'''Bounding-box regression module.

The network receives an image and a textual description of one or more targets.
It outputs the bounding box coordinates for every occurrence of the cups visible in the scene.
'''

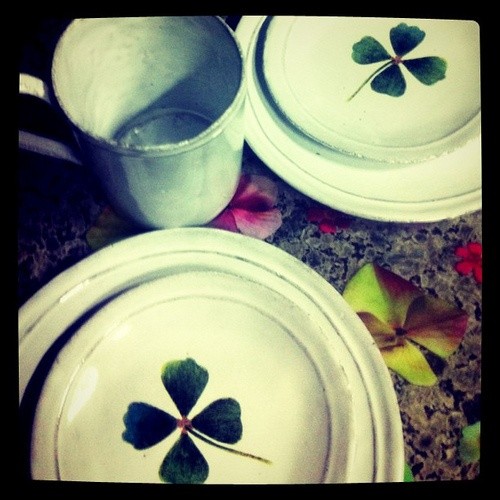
[16,18,247,230]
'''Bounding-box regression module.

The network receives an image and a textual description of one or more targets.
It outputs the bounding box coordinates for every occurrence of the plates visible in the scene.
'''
[17,227,406,484]
[235,16,481,225]
